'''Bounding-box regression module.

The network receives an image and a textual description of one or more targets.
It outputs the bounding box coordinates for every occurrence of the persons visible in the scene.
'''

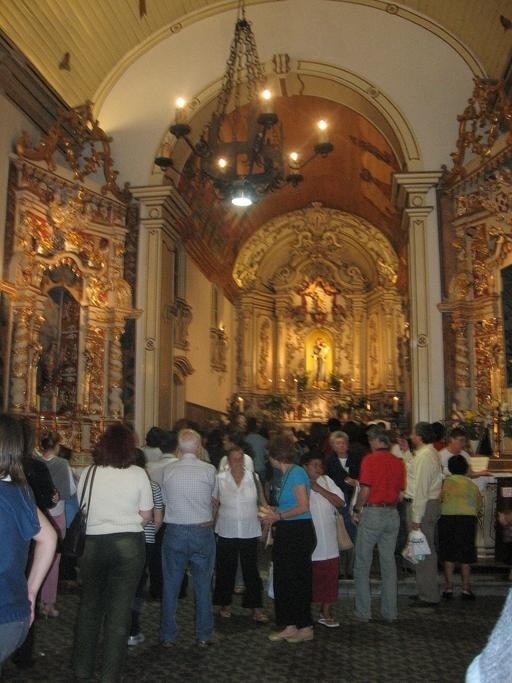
[309,337,329,381]
[3,412,511,682]
[317,340,329,362]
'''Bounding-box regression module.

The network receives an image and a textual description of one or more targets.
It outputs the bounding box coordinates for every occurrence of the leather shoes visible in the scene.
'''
[286,624,314,643]
[408,599,441,608]
[268,628,298,641]
[409,594,419,600]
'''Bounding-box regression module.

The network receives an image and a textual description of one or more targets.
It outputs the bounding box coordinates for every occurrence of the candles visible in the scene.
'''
[237,378,400,414]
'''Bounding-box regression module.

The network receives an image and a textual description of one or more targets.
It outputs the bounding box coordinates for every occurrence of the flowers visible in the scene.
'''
[461,410,479,439]
[498,402,511,437]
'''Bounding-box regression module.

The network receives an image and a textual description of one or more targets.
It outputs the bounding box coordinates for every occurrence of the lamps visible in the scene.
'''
[149,0,337,211]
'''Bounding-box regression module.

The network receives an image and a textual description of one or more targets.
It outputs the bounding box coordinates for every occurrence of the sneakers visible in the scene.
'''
[127,632,145,645]
[160,638,176,647]
[195,637,209,649]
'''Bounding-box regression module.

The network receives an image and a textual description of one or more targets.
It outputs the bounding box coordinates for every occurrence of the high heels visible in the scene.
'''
[39,602,59,623]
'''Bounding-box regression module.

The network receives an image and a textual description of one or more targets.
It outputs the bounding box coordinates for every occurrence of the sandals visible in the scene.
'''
[443,586,454,597]
[462,588,474,599]
[252,613,269,621]
[219,608,232,617]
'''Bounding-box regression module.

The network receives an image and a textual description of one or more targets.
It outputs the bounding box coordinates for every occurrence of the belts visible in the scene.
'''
[363,502,397,507]
[405,497,412,502]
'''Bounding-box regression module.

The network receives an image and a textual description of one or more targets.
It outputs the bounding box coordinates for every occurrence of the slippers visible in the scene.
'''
[317,617,341,627]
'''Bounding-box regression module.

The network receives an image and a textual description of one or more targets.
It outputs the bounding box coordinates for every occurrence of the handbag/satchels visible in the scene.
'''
[251,471,272,542]
[60,463,97,557]
[335,512,354,551]
